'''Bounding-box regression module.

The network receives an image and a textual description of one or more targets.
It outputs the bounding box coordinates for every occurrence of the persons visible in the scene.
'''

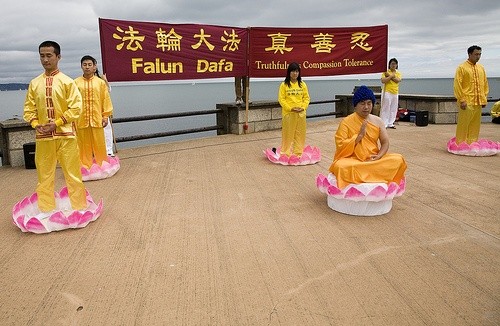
[454,46,489,146]
[329,85,408,189]
[74,55,115,171]
[380,58,402,128]
[490,101,500,124]
[23,40,87,221]
[278,62,310,158]
[235,77,252,105]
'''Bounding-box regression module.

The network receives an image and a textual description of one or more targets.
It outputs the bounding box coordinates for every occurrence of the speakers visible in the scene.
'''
[23,142,36,169]
[415,110,428,126]
